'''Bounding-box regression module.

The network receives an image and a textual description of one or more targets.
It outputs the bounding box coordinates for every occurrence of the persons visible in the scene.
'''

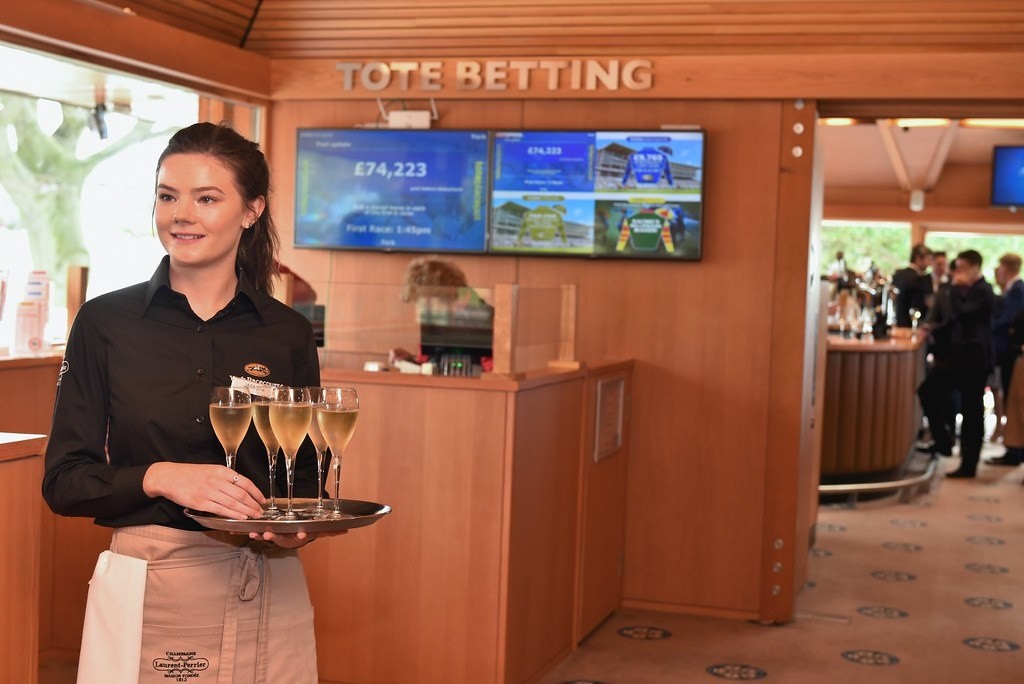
[389,258,494,373]
[892,245,1024,479]
[42,122,348,684]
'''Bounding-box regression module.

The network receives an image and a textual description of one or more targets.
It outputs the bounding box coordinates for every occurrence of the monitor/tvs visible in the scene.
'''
[293,125,702,259]
[990,146,1024,208]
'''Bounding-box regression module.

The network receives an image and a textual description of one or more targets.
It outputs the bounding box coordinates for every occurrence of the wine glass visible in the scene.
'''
[208,384,360,521]
[910,307,921,330]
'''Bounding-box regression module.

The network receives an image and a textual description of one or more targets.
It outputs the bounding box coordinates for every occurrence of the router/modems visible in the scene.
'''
[376,94,438,128]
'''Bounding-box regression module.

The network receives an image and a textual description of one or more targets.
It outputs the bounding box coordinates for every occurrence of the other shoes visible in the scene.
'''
[946,469,977,478]
[984,455,1021,466]
[914,444,953,457]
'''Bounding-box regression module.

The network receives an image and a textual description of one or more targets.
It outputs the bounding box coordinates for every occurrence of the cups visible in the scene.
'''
[839,318,863,339]
